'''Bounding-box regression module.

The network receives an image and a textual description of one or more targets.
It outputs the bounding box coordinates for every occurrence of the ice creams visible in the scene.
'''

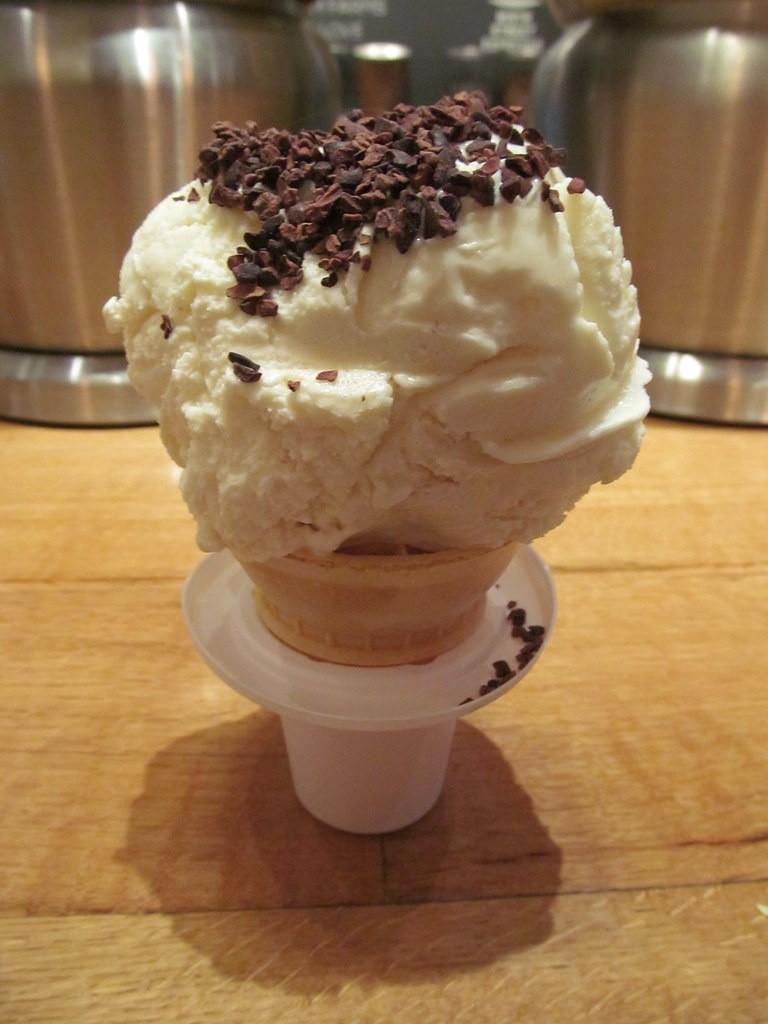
[101,89,650,668]
